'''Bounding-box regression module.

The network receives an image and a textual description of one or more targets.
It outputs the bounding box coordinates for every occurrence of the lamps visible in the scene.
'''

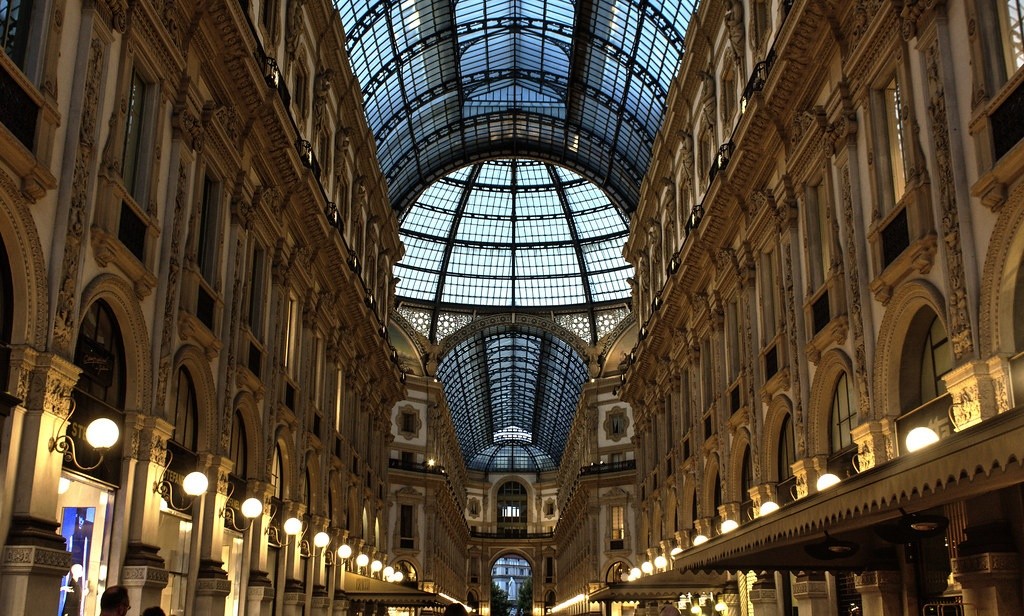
[219,471,263,531]
[906,394,967,453]
[590,461,605,466]
[720,502,757,533]
[761,479,802,515]
[48,387,118,470]
[296,521,329,557]
[323,537,352,566]
[816,446,868,490]
[259,57,404,380]
[262,499,302,547]
[370,560,382,579]
[345,553,368,576]
[611,82,748,396]
[152,434,208,510]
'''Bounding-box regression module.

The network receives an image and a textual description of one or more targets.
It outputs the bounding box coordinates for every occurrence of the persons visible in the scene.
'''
[142,606,166,616]
[508,576,515,596]
[98,584,131,616]
[61,563,83,616]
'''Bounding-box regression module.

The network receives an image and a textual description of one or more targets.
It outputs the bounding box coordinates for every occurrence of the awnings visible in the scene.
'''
[337,572,456,609]
[673,402,1022,575]
[584,570,732,604]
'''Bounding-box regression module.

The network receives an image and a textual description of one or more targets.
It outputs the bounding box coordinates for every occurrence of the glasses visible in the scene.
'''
[127,606,131,610]
